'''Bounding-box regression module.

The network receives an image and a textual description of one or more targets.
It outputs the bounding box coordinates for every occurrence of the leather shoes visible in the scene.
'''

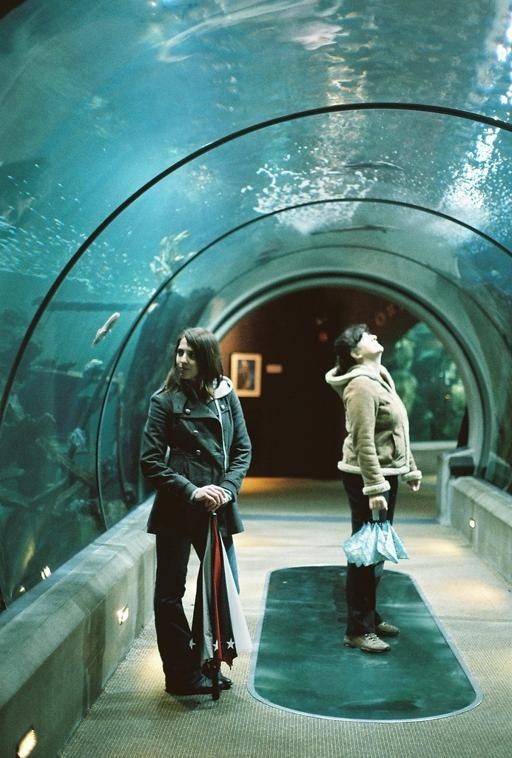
[166,666,232,693]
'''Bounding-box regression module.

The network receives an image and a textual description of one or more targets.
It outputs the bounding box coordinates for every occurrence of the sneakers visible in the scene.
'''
[374,622,400,637]
[344,631,391,653]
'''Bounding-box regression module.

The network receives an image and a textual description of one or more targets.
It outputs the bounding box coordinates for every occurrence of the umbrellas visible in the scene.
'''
[188,510,252,699]
[341,508,410,568]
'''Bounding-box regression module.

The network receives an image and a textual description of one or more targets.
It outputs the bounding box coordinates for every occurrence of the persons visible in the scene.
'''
[325,323,422,653]
[138,327,253,695]
